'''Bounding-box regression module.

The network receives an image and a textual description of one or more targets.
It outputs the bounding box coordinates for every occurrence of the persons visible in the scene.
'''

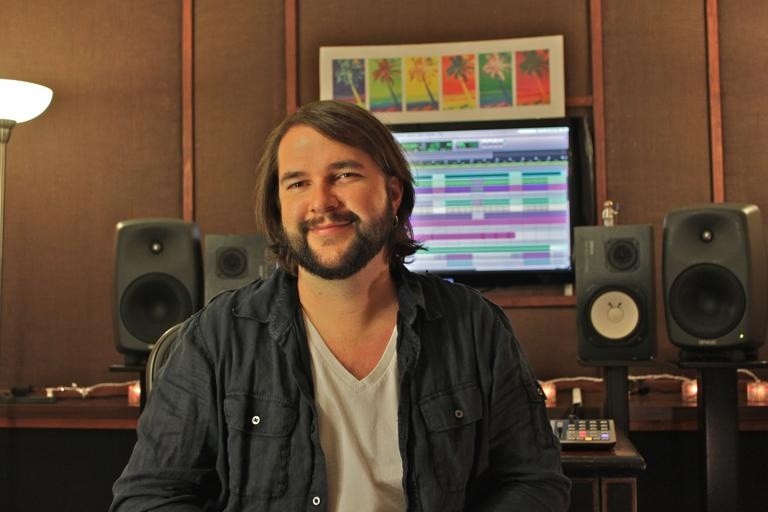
[108,101,572,511]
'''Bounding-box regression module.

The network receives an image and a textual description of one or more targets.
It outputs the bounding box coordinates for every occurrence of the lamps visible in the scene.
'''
[1,78,53,272]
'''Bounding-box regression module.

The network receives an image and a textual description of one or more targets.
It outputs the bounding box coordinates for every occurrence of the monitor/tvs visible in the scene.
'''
[382,118,585,287]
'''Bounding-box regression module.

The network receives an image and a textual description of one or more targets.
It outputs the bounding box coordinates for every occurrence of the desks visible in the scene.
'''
[1,374,768,511]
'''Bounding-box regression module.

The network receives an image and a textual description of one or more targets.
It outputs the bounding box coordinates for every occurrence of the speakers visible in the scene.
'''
[108,219,202,372]
[202,234,268,312]
[574,225,658,366]
[662,203,768,368]
